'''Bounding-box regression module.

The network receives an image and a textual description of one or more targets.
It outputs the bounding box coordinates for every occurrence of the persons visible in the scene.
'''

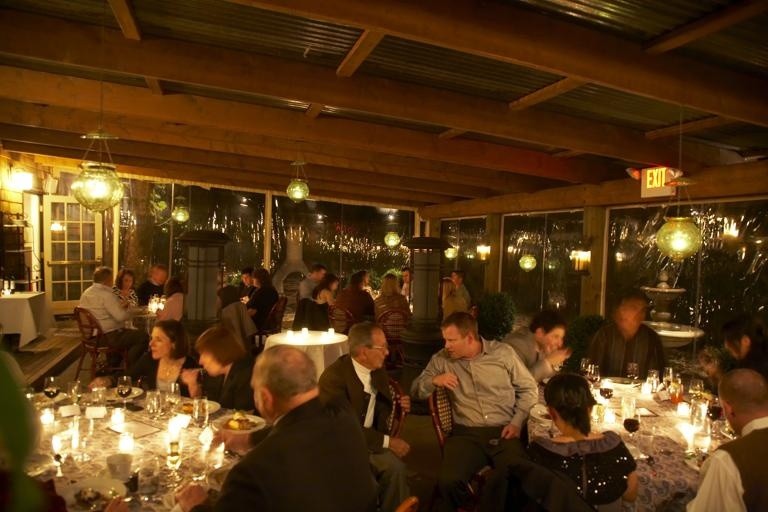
[241,269,279,331]
[441,277,467,318]
[306,274,340,331]
[217,286,257,336]
[373,273,411,320]
[127,318,201,398]
[78,265,152,380]
[712,319,766,378]
[504,309,572,383]
[237,268,253,300]
[408,311,538,511]
[451,271,472,307]
[685,368,768,512]
[528,373,635,511]
[173,343,376,512]
[318,321,410,511]
[114,269,139,306]
[299,264,327,300]
[154,277,184,321]
[358,270,373,295]
[586,289,665,381]
[401,268,411,302]
[331,274,374,336]
[194,325,254,410]
[137,265,168,306]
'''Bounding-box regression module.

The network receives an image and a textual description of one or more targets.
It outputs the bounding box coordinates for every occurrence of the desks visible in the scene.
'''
[263,329,349,384]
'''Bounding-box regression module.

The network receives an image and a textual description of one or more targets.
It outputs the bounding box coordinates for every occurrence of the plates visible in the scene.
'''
[529,405,555,424]
[36,390,67,408]
[101,385,143,404]
[212,413,267,436]
[172,400,221,418]
[64,477,128,512]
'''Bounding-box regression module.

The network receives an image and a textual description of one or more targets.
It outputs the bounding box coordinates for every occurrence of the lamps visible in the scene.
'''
[169,188,191,225]
[384,222,401,249]
[71,21,124,214]
[285,145,310,203]
[517,249,537,272]
[568,240,592,276]
[445,246,457,262]
[476,243,491,265]
[656,108,702,266]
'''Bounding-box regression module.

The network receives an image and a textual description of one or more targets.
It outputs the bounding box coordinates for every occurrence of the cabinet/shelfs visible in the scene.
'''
[0,211,44,292]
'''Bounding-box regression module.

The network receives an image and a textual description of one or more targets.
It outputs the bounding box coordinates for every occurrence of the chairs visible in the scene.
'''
[478,457,597,512]
[252,296,288,352]
[292,298,329,331]
[427,385,537,511]
[376,309,411,368]
[384,374,407,439]
[73,307,128,388]
[219,302,264,358]
[391,495,419,512]
[327,304,355,335]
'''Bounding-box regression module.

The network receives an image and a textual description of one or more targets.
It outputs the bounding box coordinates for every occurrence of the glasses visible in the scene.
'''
[365,342,388,349]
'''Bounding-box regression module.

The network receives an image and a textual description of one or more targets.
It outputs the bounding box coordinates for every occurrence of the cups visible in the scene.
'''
[641,383,651,399]
[91,386,106,409]
[621,397,636,420]
[189,446,206,480]
[39,407,54,430]
[165,437,184,483]
[106,454,133,482]
[111,408,126,425]
[118,432,133,451]
[637,433,655,460]
[138,457,161,499]
[669,384,683,409]
[647,369,660,393]
[676,402,690,417]
[203,441,225,472]
[689,379,704,398]
[146,390,162,419]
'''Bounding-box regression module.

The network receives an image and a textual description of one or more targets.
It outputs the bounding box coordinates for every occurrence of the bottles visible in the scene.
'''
[0,267,17,295]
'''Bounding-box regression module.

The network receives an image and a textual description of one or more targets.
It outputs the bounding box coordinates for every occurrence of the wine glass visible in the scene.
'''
[580,358,593,377]
[663,368,674,391]
[599,378,614,401]
[117,375,133,413]
[627,363,640,387]
[693,433,711,471]
[192,396,210,436]
[72,416,95,462]
[166,383,181,415]
[625,411,640,449]
[51,435,69,479]
[707,397,723,435]
[44,376,61,411]
[68,380,83,407]
[585,365,600,396]
[149,294,167,313]
